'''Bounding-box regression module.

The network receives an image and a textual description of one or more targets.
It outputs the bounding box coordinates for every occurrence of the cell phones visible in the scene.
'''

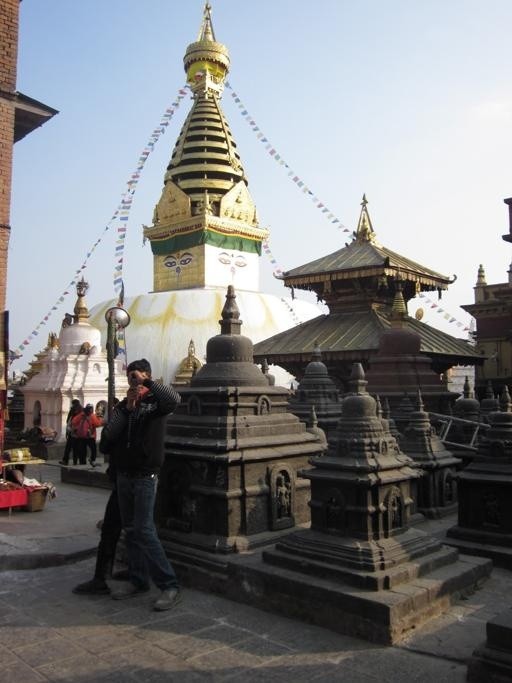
[129,376,138,388]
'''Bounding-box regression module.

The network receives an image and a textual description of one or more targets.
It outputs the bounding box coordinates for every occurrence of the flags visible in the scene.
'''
[222,76,478,339]
[9,73,194,372]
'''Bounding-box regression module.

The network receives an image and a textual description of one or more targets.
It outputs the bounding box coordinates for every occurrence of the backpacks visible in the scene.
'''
[76,415,92,438]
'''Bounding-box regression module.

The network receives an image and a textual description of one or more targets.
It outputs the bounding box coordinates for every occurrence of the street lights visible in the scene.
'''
[104,306,131,424]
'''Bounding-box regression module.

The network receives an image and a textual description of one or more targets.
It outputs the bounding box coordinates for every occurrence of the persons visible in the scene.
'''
[327,496,338,527]
[68,425,131,595]
[275,474,290,518]
[99,357,183,611]
[189,402,197,414]
[57,398,82,465]
[261,400,268,414]
[71,402,104,467]
[391,497,400,524]
[445,483,452,500]
[177,337,202,372]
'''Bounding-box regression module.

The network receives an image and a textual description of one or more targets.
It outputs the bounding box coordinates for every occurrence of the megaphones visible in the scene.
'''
[104,307,132,328]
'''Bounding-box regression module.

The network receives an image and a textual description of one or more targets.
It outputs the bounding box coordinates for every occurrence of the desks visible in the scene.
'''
[1,455,47,515]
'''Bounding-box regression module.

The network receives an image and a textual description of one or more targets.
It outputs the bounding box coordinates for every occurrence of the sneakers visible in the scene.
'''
[153,589,183,611]
[88,458,95,467]
[59,461,68,465]
[72,580,114,594]
[113,570,130,582]
[111,585,149,600]
